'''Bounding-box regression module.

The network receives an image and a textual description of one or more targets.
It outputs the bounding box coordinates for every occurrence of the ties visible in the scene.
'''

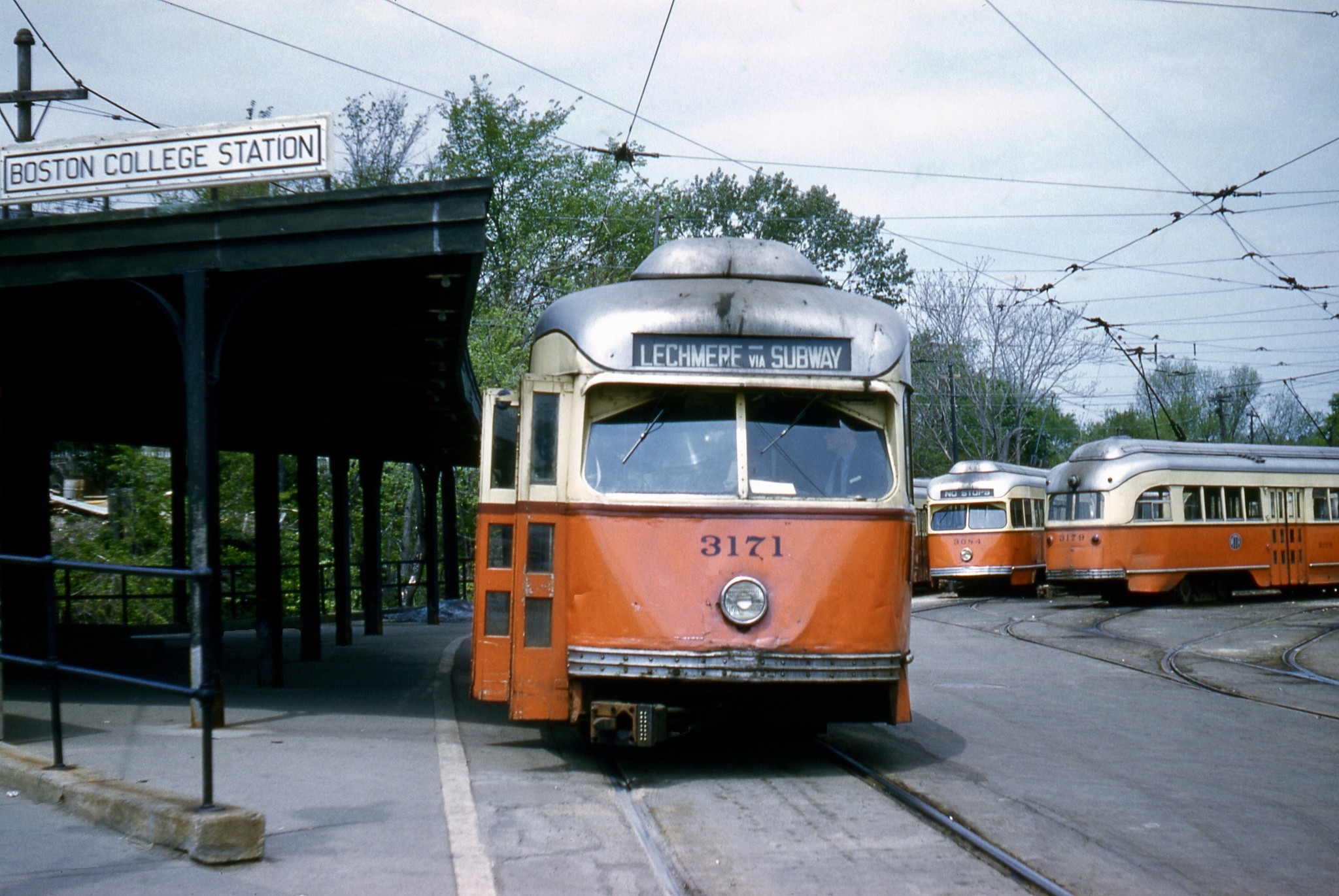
[832,459,842,496]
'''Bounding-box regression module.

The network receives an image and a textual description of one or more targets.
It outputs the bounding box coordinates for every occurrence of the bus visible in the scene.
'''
[913,478,943,593]
[469,235,917,757]
[1043,435,1339,606]
[926,460,1093,598]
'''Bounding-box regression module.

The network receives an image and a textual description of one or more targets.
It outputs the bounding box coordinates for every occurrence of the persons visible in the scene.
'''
[807,415,890,497]
